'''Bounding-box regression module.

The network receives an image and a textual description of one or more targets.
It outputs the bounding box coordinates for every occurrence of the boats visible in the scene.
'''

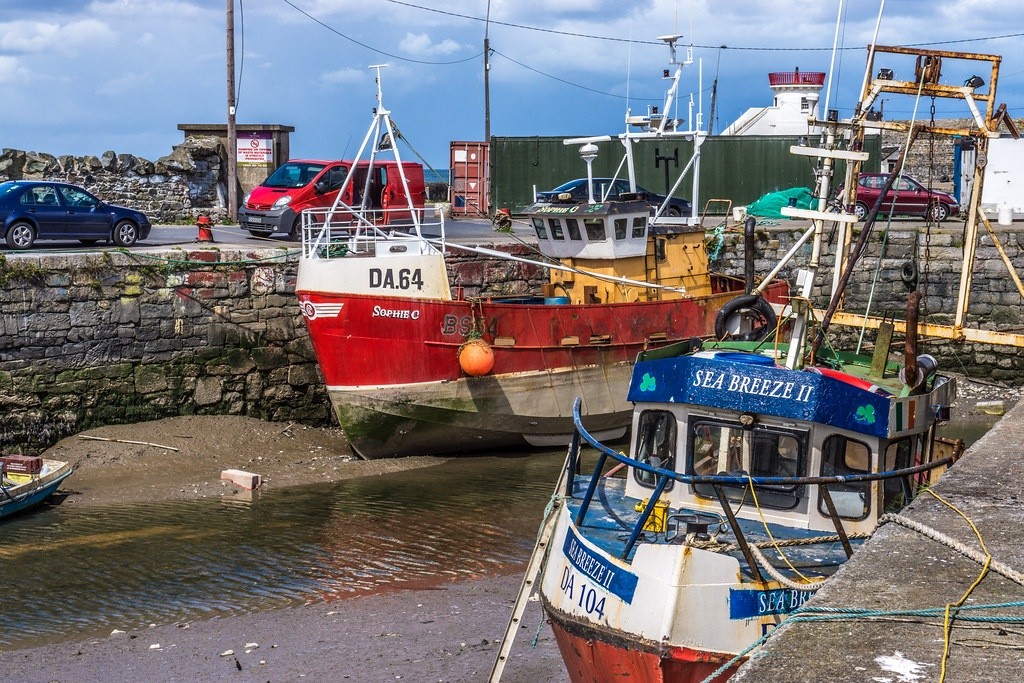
[0,455,74,521]
[489,107,965,683]
[295,58,791,460]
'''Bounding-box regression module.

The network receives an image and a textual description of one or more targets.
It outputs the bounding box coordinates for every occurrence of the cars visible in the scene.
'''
[536,178,693,218]
[0,180,153,253]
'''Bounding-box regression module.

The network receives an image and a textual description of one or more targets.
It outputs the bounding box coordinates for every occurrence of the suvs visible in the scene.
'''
[836,172,961,222]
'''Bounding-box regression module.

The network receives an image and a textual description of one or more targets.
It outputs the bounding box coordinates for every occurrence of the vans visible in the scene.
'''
[238,158,425,242]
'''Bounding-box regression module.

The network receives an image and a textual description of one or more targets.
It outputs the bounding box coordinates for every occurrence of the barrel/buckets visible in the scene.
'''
[545,297,568,304]
